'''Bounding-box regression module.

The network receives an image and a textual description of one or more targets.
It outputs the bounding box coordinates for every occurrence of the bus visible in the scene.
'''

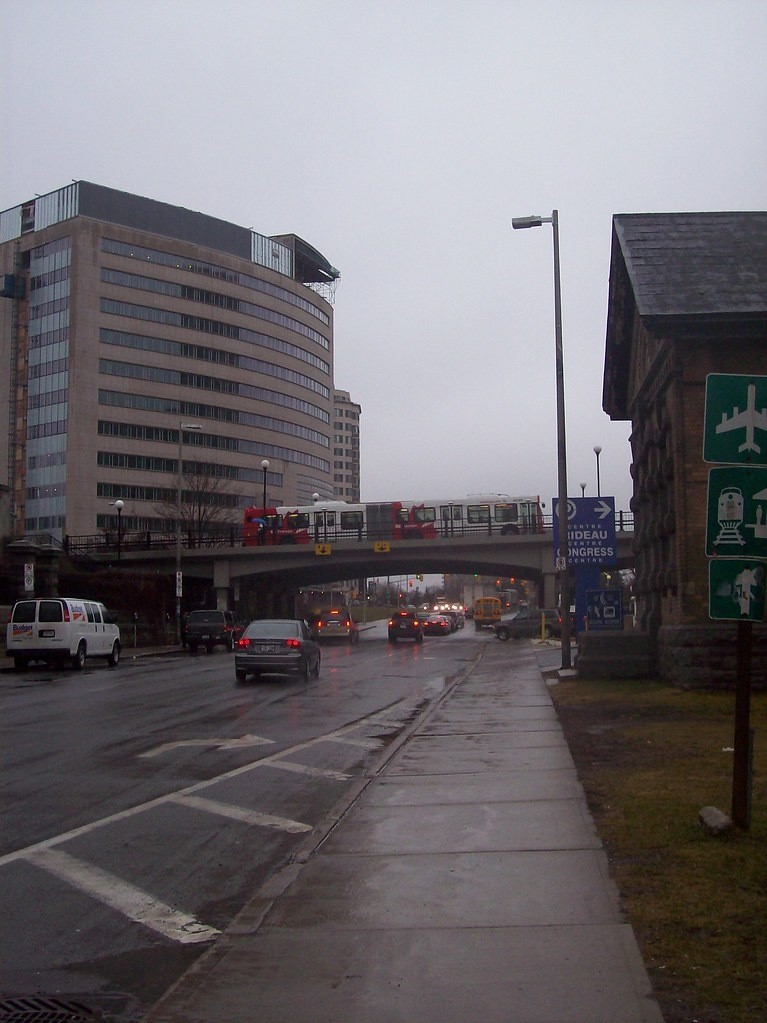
[242,494,545,549]
[475,597,503,629]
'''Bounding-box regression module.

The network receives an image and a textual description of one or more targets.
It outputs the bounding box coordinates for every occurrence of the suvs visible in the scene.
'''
[186,609,239,655]
[388,610,425,643]
[492,608,575,642]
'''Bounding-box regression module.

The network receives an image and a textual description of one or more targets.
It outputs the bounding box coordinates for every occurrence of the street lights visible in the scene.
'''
[259,458,271,509]
[173,421,204,641]
[511,210,571,667]
[115,500,125,568]
[580,482,587,498]
[593,445,603,500]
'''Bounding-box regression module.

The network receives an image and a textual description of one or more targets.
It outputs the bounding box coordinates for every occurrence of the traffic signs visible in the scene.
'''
[553,496,616,575]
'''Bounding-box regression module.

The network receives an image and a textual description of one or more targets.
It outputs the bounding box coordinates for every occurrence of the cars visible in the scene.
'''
[316,609,359,643]
[235,619,322,683]
[408,602,465,630]
[423,614,451,635]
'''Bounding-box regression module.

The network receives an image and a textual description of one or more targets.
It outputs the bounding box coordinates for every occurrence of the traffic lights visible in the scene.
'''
[524,588,529,596]
[474,574,480,581]
[416,574,421,579]
[409,581,412,587]
[399,593,402,598]
[510,578,515,585]
[421,576,423,582]
[496,580,501,588]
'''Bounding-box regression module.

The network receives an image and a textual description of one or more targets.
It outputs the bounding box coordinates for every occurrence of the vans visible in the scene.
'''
[5,597,123,673]
